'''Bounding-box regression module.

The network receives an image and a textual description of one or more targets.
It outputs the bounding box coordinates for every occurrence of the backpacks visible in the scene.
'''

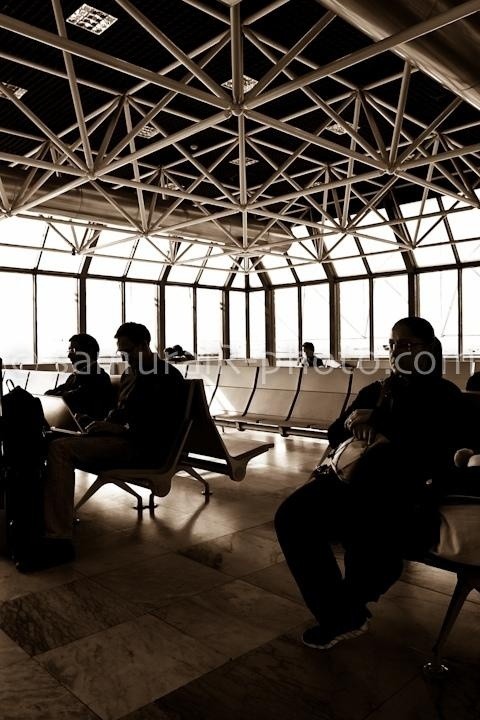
[1,385,53,556]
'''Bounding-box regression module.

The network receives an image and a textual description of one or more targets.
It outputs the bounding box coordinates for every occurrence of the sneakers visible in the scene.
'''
[301,612,370,650]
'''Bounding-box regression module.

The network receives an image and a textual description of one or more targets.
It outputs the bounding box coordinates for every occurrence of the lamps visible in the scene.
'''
[0,3,361,192]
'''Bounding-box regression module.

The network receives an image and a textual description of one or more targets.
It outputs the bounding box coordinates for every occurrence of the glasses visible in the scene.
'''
[388,339,415,353]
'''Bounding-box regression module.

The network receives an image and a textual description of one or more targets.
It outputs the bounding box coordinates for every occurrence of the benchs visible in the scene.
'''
[0,358,480,674]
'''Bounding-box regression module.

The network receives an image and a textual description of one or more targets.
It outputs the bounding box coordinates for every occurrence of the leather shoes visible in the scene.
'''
[15,537,78,574]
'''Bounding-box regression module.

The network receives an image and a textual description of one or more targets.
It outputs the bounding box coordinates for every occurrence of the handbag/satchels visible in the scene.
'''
[311,432,397,485]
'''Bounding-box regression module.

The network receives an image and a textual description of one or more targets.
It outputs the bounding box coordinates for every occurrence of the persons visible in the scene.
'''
[297,342,324,368]
[44,322,195,540]
[274,317,480,650]
